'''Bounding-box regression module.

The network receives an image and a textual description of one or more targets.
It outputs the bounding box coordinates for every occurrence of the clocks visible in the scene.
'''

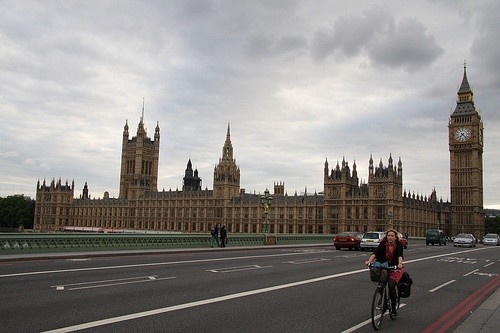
[454,127,471,142]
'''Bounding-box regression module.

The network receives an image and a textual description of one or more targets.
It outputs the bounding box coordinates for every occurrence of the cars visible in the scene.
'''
[446,234,481,244]
[359,231,386,252]
[397,232,409,249]
[453,233,477,248]
[482,233,500,246]
[333,231,363,251]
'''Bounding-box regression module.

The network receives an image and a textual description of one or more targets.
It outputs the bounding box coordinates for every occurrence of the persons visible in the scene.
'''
[365,230,403,318]
[211,224,227,248]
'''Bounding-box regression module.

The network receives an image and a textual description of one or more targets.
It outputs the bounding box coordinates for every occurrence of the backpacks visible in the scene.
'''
[211,228,215,235]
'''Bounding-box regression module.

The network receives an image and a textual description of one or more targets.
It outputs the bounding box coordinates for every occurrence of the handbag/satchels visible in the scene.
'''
[398,277,413,298]
[371,261,388,281]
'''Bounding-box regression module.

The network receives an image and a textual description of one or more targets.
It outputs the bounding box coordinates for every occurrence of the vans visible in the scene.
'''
[425,229,448,246]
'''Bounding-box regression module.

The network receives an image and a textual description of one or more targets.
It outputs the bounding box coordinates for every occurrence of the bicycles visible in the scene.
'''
[366,262,404,331]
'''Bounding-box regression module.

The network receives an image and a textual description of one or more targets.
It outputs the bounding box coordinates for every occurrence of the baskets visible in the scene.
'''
[370,265,391,283]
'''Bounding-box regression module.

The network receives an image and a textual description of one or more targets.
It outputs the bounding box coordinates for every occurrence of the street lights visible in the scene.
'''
[259,187,274,233]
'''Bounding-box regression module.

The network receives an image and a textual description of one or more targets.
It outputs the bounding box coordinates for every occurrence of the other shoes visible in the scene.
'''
[390,310,397,316]
[378,299,382,305]
